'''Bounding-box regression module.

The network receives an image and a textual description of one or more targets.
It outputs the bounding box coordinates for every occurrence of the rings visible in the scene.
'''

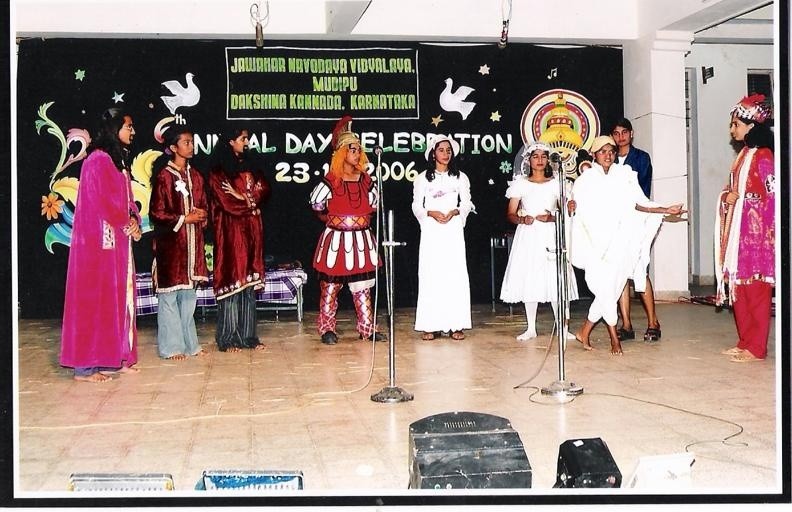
[728,201,730,204]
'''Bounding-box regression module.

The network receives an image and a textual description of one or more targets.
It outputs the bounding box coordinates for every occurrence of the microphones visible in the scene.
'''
[372,146,383,158]
[550,153,563,163]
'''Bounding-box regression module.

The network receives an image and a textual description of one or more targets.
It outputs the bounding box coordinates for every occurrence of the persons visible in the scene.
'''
[716,94,776,363]
[566,135,687,354]
[411,135,476,341]
[610,118,663,343]
[309,131,388,345]
[498,145,578,342]
[205,122,268,354]
[150,123,210,361]
[59,108,143,383]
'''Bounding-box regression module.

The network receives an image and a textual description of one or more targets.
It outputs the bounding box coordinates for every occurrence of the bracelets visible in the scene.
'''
[454,209,459,215]
[427,211,430,216]
[520,216,522,224]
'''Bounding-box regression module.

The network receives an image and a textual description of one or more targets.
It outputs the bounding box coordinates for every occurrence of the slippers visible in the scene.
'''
[644,321,661,341]
[730,351,765,362]
[449,330,464,339]
[616,328,637,340]
[422,331,442,341]
[723,348,744,355]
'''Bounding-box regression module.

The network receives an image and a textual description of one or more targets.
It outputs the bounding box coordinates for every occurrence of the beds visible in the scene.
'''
[136,260,308,322]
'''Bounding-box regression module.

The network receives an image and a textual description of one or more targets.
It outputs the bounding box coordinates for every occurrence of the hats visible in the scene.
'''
[332,116,360,150]
[732,94,771,123]
[591,136,616,152]
[425,134,460,160]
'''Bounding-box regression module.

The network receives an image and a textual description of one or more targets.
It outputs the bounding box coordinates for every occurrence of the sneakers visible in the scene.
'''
[321,331,339,347]
[360,332,388,342]
